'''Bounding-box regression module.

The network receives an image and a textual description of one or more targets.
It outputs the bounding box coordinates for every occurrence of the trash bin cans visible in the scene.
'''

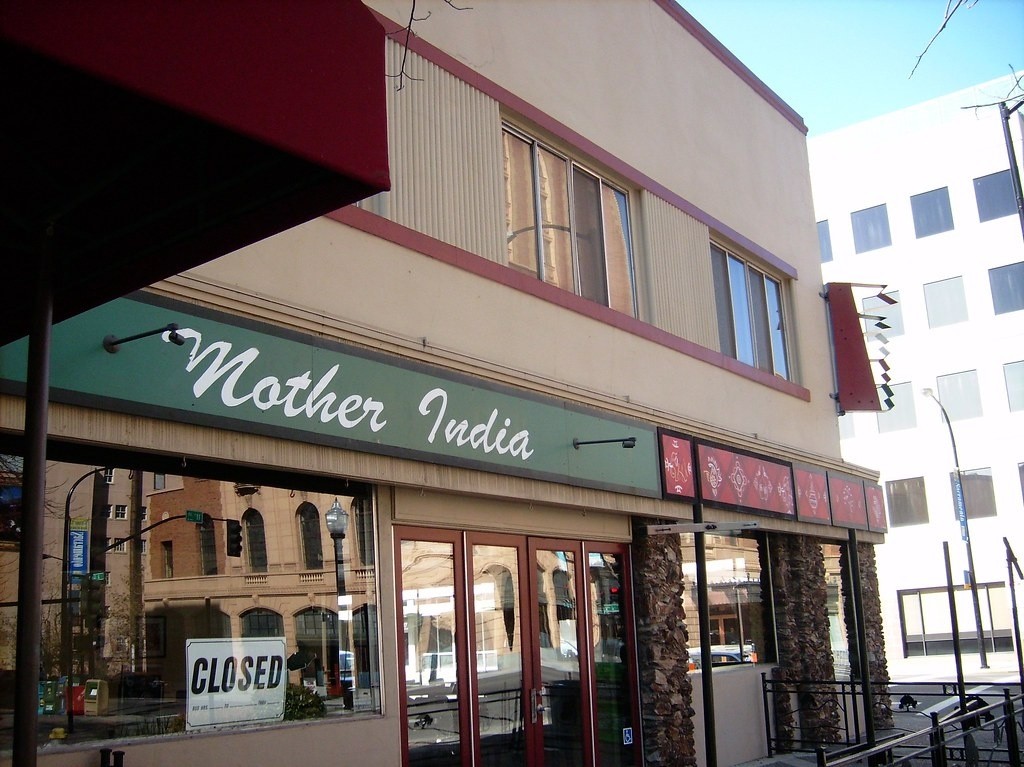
[63,685,85,714]
[549,679,581,744]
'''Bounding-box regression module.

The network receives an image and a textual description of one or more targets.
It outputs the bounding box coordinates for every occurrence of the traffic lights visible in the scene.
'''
[226,518,243,558]
[79,572,103,616]
[608,580,619,605]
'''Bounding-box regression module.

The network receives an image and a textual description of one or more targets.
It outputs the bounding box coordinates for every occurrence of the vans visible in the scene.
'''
[686,643,754,669]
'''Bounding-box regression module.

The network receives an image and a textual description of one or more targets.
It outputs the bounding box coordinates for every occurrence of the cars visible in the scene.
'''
[337,650,353,694]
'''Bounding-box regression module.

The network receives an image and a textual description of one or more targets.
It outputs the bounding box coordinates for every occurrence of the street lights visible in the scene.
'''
[919,386,989,668]
